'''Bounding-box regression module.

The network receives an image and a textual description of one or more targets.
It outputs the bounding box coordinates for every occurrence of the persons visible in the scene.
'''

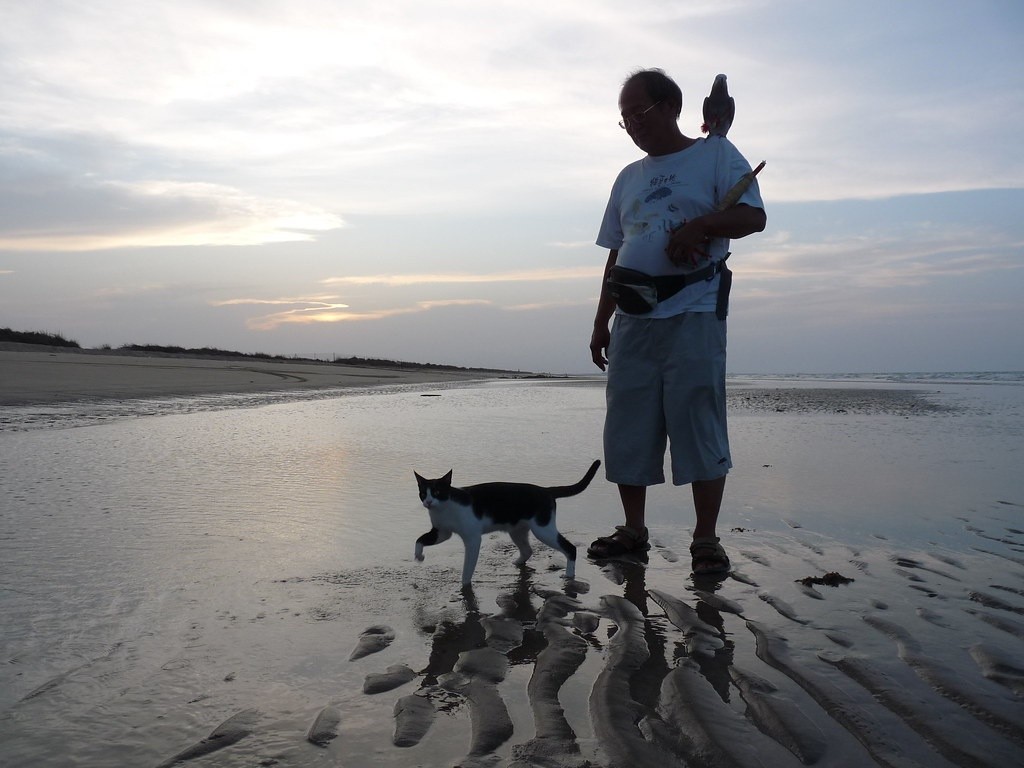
[588,68,768,580]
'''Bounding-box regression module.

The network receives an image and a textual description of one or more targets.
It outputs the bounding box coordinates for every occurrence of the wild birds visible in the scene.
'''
[699,73,735,146]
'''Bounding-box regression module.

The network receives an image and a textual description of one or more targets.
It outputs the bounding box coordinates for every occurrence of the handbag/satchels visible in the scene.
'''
[605,262,722,316]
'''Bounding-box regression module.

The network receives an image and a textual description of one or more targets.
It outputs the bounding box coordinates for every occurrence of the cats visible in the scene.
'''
[412,458,603,586]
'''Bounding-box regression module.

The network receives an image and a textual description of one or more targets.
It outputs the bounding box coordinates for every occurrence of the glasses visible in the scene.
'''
[619,101,661,129]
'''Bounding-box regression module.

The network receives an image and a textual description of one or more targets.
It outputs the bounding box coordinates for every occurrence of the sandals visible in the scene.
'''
[689,537,731,573]
[588,525,651,558]
